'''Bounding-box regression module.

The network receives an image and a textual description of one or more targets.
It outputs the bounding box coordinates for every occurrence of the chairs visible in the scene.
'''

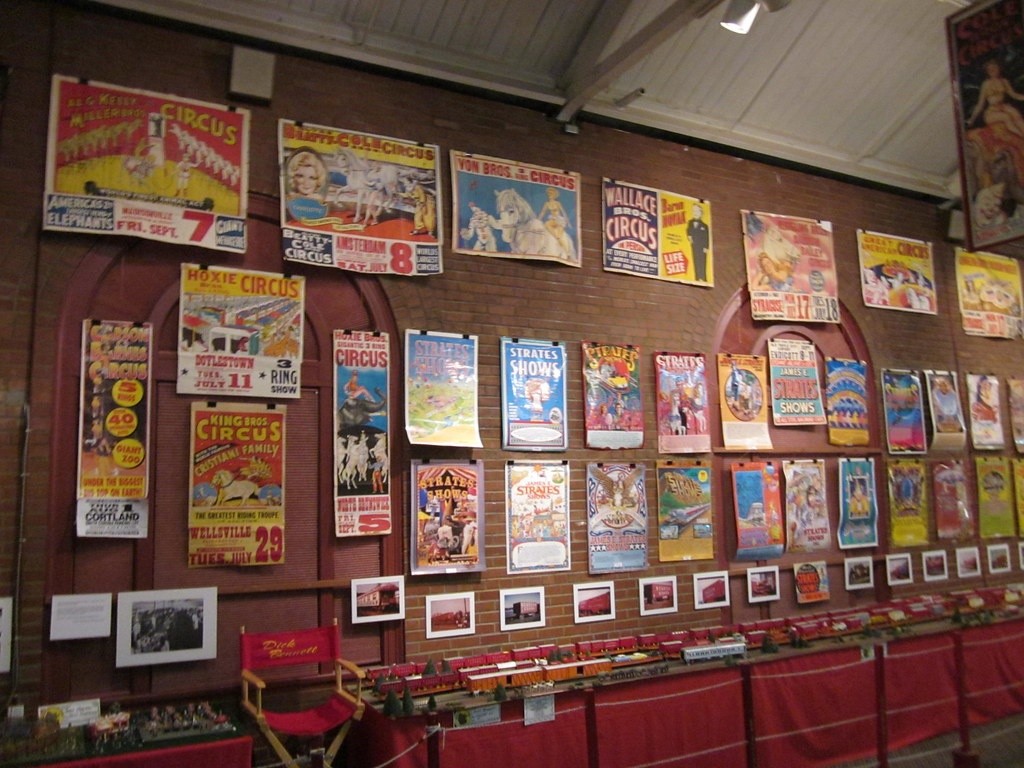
[242,617,365,768]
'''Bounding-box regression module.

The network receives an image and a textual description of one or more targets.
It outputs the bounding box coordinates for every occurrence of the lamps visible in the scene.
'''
[720,0,789,35]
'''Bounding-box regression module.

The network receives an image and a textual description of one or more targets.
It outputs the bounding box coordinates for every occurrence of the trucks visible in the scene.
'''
[512,602,540,620]
[644,583,673,604]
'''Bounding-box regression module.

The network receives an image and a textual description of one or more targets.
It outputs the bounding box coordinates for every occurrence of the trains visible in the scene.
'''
[357,583,399,613]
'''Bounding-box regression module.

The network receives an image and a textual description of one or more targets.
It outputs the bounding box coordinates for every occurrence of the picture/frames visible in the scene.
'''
[747,564,781,603]
[499,586,546,630]
[693,570,730,610]
[425,591,475,639]
[638,575,678,617]
[946,0,1024,252]
[350,574,406,624]
[573,580,617,623]
[115,586,218,670]
[843,541,1024,591]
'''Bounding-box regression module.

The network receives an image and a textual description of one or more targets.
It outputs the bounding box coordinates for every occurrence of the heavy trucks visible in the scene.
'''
[579,591,611,615]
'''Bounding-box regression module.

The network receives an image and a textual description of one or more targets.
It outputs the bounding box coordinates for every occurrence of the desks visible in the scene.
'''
[42,732,253,768]
[356,618,1024,768]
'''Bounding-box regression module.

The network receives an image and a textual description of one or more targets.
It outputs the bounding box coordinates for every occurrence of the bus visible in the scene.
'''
[679,642,747,666]
